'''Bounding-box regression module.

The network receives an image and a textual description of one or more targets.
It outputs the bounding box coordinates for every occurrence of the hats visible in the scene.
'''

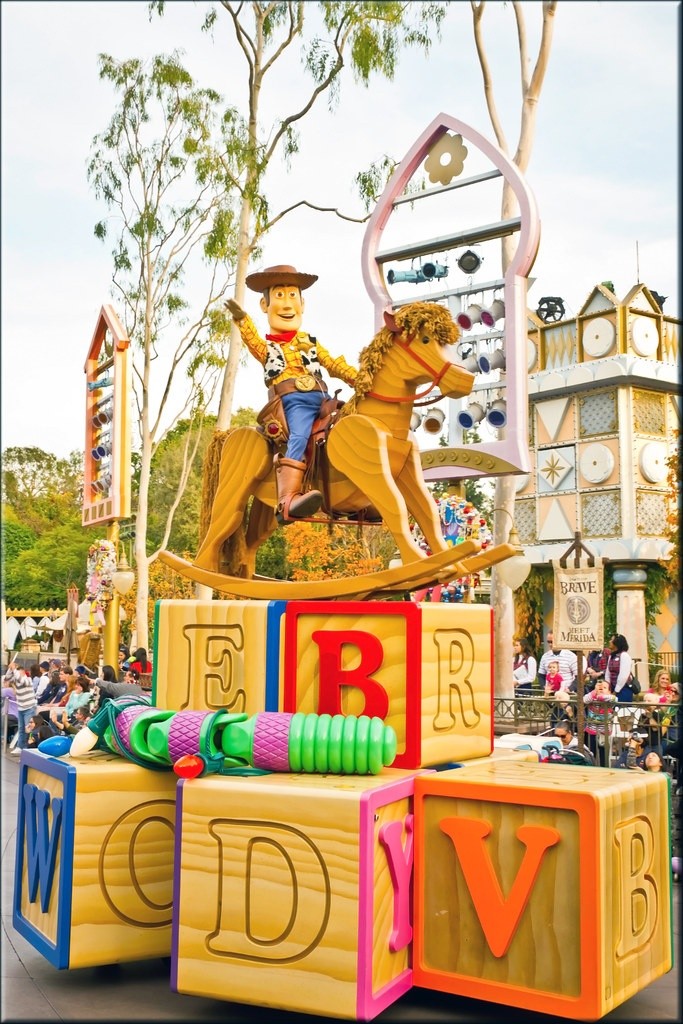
[40,661,49,671]
[669,682,682,696]
[39,711,49,722]
[75,666,86,674]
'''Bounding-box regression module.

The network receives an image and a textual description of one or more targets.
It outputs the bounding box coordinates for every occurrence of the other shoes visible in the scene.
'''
[11,747,22,756]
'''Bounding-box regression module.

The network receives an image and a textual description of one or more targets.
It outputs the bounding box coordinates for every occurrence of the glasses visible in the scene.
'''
[671,690,680,695]
[547,640,553,643]
[555,732,568,739]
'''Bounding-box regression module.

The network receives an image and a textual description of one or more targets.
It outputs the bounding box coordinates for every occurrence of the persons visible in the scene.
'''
[1,644,152,755]
[224,266,360,526]
[513,638,537,717]
[537,631,681,777]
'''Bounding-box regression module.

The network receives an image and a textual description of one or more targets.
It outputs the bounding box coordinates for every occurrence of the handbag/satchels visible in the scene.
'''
[598,734,610,747]
[630,677,640,694]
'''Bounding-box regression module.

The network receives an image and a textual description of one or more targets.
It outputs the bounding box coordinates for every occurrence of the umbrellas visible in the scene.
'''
[60,598,79,666]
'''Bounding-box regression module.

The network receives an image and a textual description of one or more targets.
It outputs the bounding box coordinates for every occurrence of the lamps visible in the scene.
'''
[488,398,506,427]
[387,269,421,283]
[456,250,481,274]
[458,402,485,427]
[478,350,507,372]
[461,355,479,376]
[457,304,484,330]
[424,408,445,433]
[422,263,448,282]
[480,297,505,327]
